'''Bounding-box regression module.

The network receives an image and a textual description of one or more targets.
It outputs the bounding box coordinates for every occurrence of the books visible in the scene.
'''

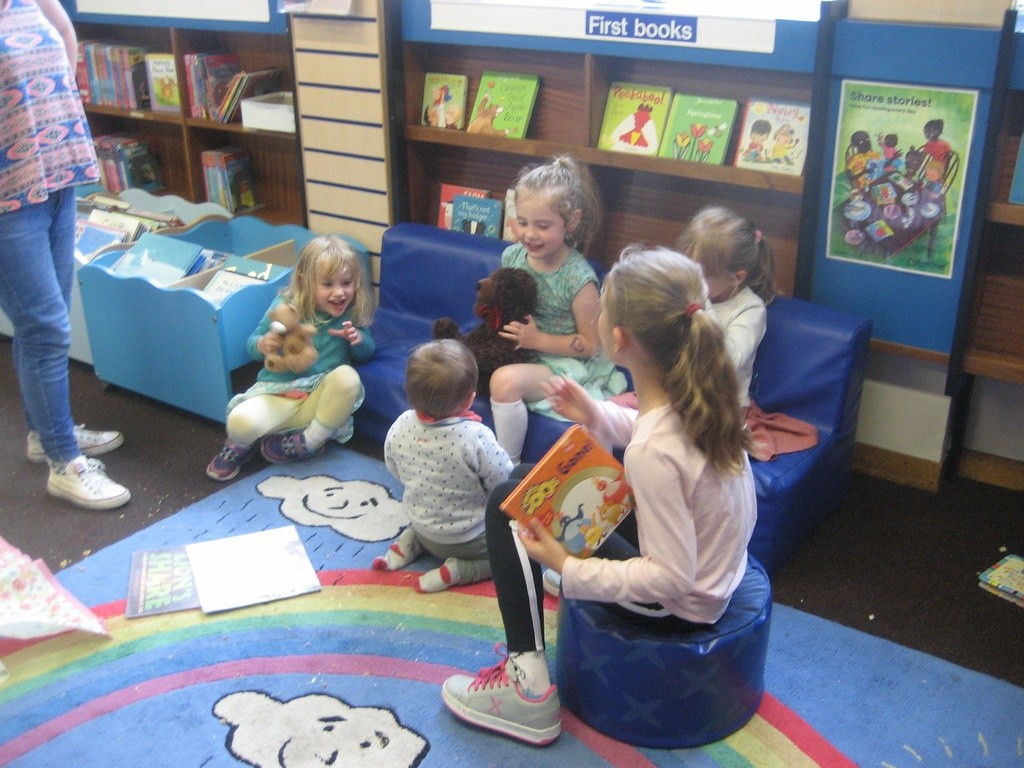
[468,69,541,139]
[75,37,182,113]
[438,183,490,230]
[185,525,322,615]
[108,231,233,301]
[420,70,467,130]
[658,92,739,165]
[202,251,289,309]
[183,49,282,124]
[733,95,812,175]
[73,195,187,267]
[502,188,522,244]
[126,545,200,620]
[499,425,637,560]
[199,145,258,211]
[452,194,503,238]
[595,81,673,156]
[90,132,167,194]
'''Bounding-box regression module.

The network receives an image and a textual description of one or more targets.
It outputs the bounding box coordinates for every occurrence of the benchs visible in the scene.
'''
[350,219,876,569]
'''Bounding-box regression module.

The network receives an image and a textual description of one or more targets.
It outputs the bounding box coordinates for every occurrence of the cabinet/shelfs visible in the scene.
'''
[65,0,1024,496]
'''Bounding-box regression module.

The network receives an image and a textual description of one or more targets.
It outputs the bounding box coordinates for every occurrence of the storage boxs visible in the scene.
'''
[240,89,298,135]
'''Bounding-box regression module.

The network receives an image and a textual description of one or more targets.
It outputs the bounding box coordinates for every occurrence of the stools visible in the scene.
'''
[552,553,774,751]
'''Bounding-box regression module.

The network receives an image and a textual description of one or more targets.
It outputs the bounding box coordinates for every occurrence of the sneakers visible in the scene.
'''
[46,455,131,510]
[206,437,255,482]
[441,642,561,746]
[26,424,125,464]
[259,425,327,464]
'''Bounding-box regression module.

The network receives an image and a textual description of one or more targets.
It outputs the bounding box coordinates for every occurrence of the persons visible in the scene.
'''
[204,235,376,482]
[367,339,517,596]
[0,0,132,512]
[603,206,768,419]
[482,155,629,471]
[442,246,758,748]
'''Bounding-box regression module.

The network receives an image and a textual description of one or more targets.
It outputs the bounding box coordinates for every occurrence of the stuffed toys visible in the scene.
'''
[433,267,538,396]
[265,304,320,373]
